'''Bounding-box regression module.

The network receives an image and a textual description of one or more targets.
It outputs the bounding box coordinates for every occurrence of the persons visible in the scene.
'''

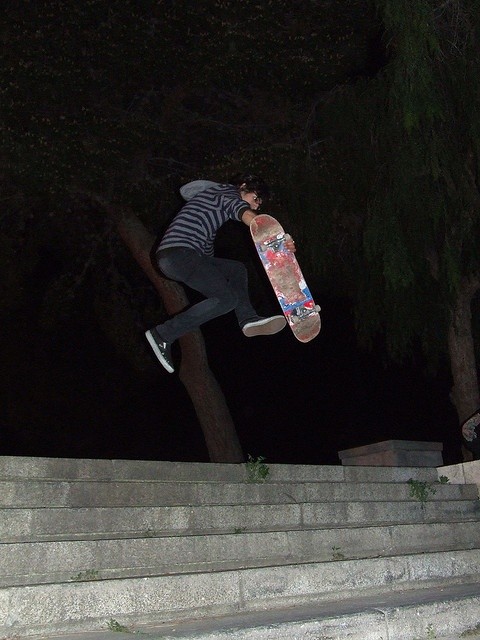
[144,174,297,373]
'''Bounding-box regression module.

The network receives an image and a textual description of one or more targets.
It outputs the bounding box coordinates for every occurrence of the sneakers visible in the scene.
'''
[145,327,175,374]
[239,314,287,337]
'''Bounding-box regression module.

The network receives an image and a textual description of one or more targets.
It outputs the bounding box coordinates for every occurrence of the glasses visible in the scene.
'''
[252,190,264,212]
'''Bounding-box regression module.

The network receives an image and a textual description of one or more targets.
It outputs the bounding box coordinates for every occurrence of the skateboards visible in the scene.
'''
[250,214,321,343]
